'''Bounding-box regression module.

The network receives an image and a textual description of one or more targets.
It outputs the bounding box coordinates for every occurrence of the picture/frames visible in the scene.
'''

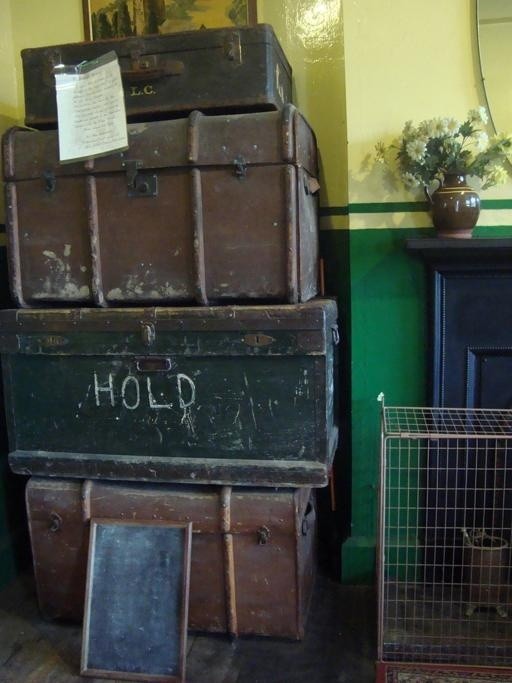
[82,0,258,41]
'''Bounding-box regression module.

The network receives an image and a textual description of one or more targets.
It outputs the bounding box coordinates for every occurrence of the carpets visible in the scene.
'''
[376,662,512,683]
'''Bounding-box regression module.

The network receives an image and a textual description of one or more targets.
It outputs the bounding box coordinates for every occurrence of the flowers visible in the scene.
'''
[372,106,512,189]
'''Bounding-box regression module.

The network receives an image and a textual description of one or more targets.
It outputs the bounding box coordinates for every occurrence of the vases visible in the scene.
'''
[423,172,481,239]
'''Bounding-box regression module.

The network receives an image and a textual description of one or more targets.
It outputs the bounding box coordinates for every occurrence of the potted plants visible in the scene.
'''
[462,527,510,618]
